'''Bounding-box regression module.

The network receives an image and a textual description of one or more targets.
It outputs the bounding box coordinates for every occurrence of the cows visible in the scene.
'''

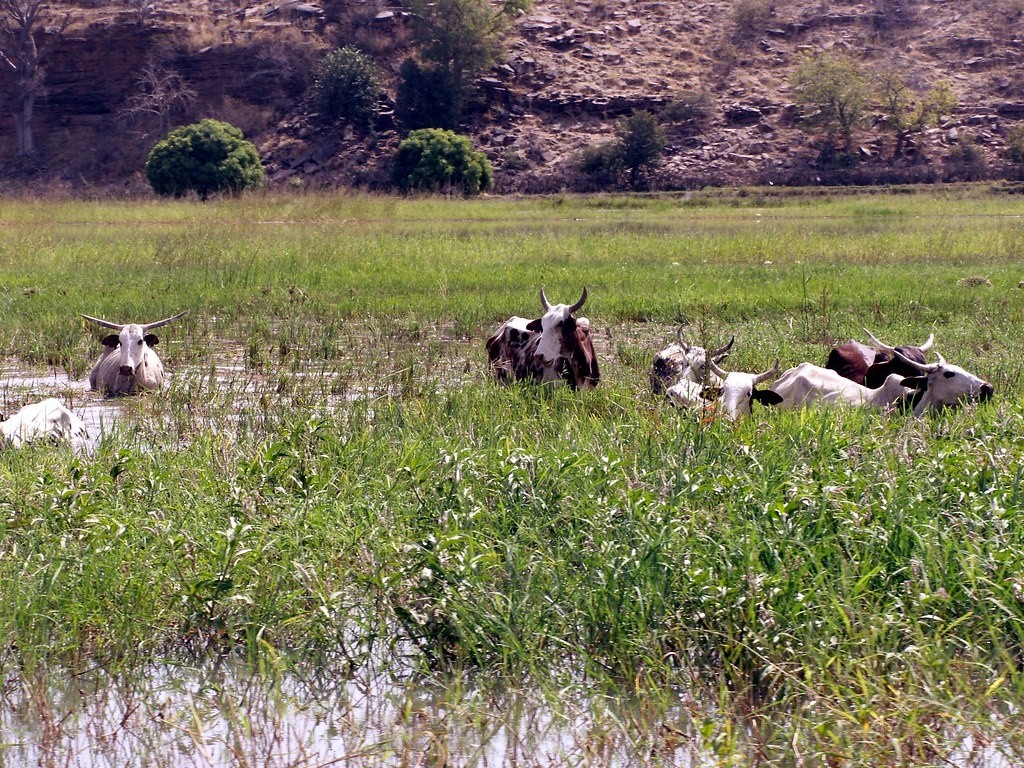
[485,283,602,395]
[652,323,994,419]
[79,311,191,394]
